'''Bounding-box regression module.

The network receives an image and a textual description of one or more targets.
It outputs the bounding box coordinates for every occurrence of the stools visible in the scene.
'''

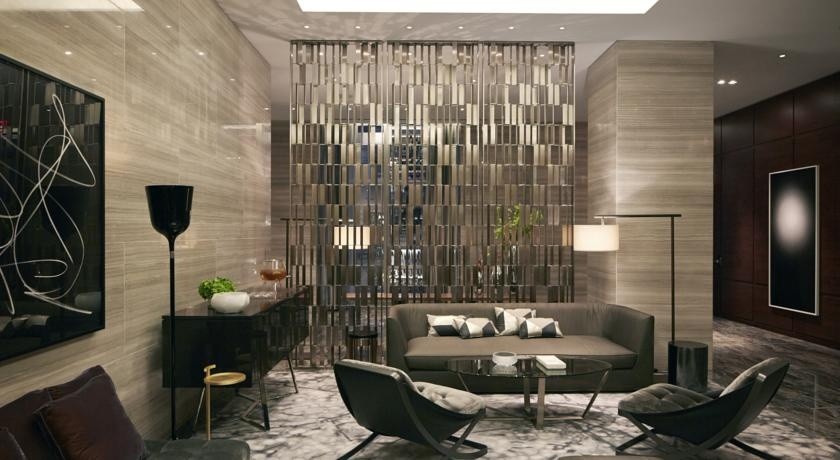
[348,329,379,364]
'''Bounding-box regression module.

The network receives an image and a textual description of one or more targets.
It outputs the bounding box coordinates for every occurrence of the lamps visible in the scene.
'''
[570,213,683,394]
[142,184,193,442]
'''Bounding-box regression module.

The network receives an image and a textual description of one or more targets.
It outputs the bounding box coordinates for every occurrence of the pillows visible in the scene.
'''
[425,313,474,337]
[493,308,536,336]
[516,316,564,339]
[453,317,500,339]
[30,371,154,460]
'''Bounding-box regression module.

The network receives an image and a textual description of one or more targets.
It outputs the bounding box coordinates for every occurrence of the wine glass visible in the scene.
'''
[260,259,286,304]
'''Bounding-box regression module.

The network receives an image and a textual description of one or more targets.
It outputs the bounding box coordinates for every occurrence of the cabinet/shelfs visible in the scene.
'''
[161,285,310,441]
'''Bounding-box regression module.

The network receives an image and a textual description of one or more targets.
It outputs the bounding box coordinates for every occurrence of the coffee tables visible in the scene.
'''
[439,356,614,429]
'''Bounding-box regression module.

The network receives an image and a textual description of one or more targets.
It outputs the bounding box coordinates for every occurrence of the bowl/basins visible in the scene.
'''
[492,351,517,366]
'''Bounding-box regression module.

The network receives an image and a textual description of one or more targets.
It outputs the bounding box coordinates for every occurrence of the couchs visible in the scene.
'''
[332,358,489,460]
[0,364,252,460]
[617,351,790,460]
[386,301,655,394]
